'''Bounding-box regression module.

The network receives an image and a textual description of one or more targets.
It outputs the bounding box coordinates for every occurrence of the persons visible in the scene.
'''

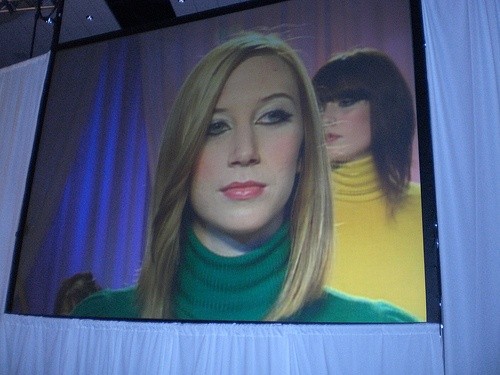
[51,271,102,316]
[311,48,427,322]
[70,32,420,324]
